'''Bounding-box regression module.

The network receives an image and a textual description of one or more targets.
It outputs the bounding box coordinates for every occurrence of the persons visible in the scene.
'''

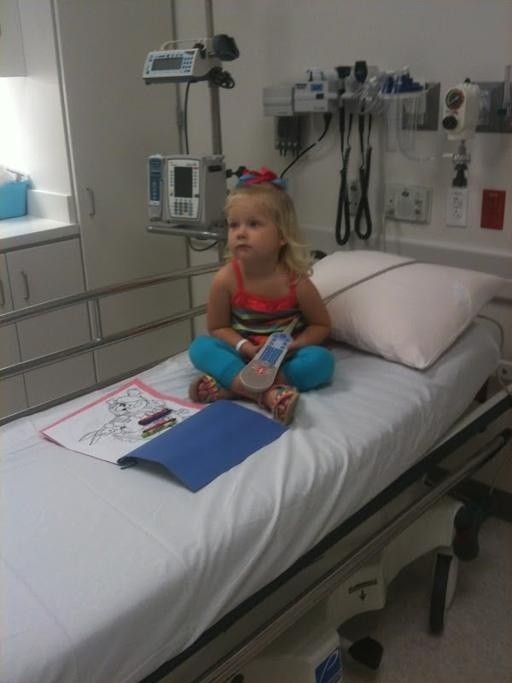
[188,166,337,427]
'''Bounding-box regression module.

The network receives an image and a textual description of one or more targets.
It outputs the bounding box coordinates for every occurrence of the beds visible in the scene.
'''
[0,319,511,683]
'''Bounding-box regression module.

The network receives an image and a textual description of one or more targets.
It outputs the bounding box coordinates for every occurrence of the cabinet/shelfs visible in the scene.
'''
[18,2,195,387]
[0,236,97,420]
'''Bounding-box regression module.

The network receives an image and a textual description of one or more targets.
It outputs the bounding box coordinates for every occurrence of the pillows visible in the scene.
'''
[309,250,503,370]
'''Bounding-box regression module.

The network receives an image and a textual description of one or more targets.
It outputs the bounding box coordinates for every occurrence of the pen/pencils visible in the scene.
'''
[139,409,176,438]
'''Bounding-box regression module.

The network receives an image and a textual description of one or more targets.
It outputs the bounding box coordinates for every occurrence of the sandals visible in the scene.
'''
[188,372,225,404]
[256,383,300,426]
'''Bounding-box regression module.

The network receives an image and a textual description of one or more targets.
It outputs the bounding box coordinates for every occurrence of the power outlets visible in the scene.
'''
[446,189,468,227]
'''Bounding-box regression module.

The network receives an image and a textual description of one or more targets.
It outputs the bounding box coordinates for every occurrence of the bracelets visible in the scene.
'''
[236,338,250,352]
